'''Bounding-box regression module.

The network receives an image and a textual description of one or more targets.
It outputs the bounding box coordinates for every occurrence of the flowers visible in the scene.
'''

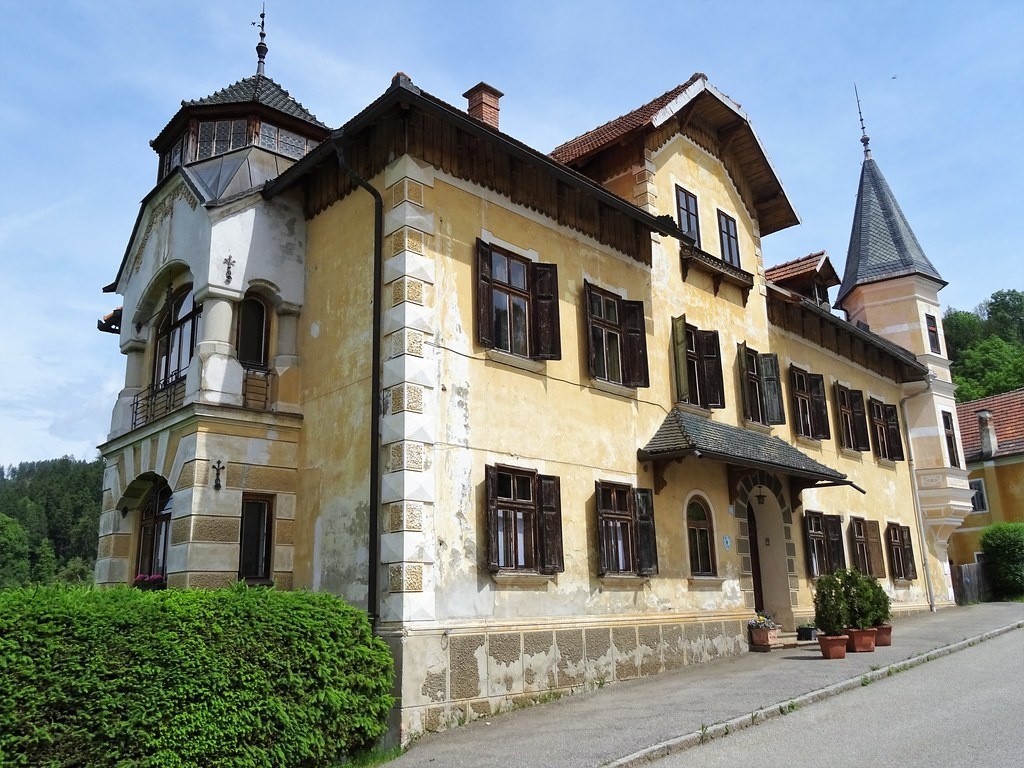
[748,610,776,629]
[135,574,163,581]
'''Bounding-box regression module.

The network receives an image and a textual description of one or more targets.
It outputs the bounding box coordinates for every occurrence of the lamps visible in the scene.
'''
[754,487,767,504]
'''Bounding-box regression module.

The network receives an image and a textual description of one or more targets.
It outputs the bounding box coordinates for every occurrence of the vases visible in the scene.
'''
[750,628,777,645]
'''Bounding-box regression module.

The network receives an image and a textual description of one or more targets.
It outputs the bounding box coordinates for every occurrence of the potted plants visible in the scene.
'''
[798,622,816,641]
[813,566,893,659]
